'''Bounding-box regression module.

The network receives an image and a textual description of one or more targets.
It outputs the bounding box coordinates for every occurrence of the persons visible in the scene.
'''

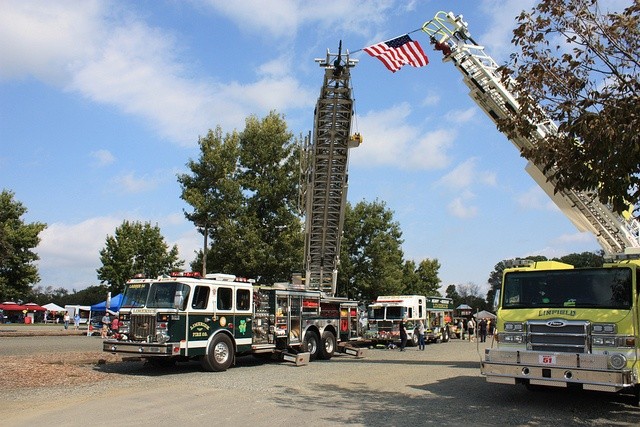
[112,316,118,339]
[100,312,110,338]
[400,316,408,351]
[418,319,425,350]
[63,311,70,329]
[453,315,495,344]
[73,313,81,332]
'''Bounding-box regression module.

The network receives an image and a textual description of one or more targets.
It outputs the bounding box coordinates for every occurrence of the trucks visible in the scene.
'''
[364,294,458,346]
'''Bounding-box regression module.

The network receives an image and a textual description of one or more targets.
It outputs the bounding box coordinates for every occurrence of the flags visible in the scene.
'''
[363,34,429,72]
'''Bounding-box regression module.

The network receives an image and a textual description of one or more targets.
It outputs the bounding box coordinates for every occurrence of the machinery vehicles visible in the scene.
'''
[103,40,364,371]
[423,11,640,392]
